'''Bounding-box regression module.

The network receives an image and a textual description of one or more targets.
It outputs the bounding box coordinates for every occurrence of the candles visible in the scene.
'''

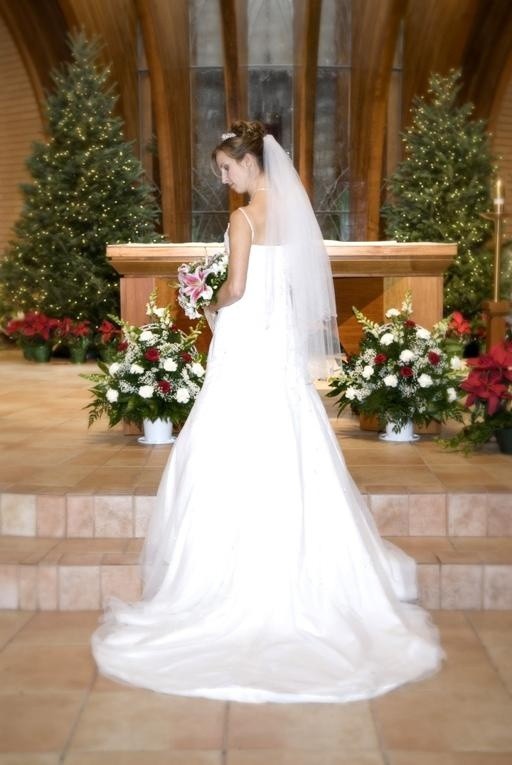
[494,179,504,204]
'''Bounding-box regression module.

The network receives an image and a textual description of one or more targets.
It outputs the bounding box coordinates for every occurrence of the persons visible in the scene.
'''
[95,119,443,703]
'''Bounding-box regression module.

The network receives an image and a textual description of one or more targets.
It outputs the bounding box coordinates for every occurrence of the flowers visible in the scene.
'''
[322,296,510,431]
[81,288,207,425]
[176,252,229,320]
[12,309,127,347]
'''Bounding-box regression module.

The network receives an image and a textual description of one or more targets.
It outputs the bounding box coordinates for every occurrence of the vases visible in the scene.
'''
[380,418,416,442]
[493,426,511,456]
[142,418,173,442]
[17,341,120,363]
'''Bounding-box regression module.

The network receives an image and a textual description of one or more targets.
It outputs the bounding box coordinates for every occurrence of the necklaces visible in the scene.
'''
[248,187,273,205]
